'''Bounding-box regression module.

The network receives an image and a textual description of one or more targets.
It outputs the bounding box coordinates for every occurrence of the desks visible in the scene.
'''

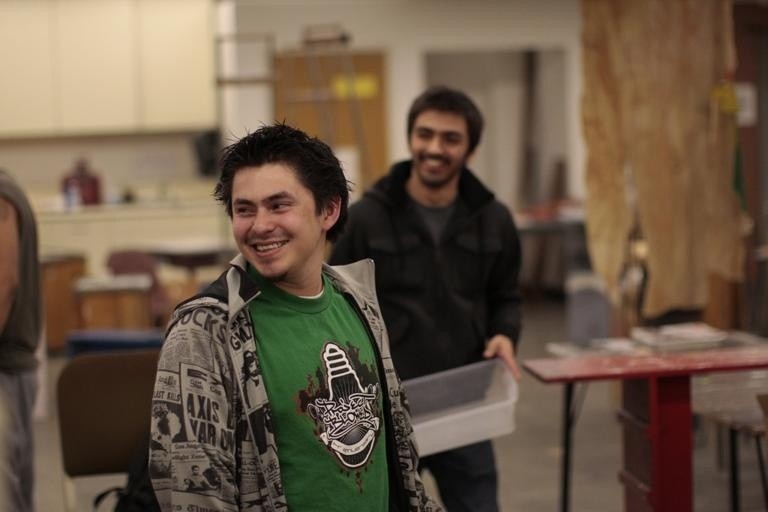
[520,340,768,512]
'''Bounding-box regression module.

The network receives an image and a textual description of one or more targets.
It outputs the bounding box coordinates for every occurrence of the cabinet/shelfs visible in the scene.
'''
[0,1,224,144]
[23,202,229,258]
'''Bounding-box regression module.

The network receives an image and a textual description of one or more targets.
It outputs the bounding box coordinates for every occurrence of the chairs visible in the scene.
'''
[52,333,173,510]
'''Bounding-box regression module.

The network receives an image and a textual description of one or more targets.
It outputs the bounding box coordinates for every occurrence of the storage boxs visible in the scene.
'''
[398,354,523,460]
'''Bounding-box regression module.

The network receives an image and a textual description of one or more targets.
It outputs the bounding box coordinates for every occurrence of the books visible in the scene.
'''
[547,320,748,362]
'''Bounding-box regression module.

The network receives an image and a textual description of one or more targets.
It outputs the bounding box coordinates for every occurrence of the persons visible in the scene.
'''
[148,115,445,511]
[1,166,101,511]
[327,85,523,510]
